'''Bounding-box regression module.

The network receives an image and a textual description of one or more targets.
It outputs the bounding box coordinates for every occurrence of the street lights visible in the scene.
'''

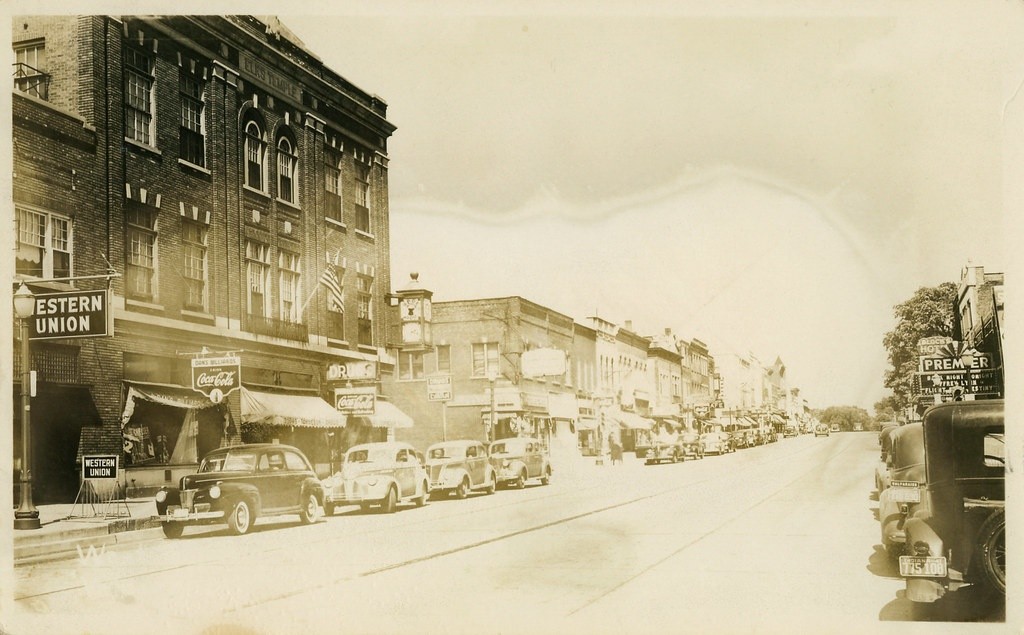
[727,403,733,432]
[488,367,497,441]
[685,396,692,426]
[14,282,42,530]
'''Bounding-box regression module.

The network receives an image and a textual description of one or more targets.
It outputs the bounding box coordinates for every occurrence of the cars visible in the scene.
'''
[731,427,778,449]
[676,434,705,460]
[423,439,497,500]
[645,436,686,465]
[831,424,840,433]
[320,441,432,517]
[898,400,1005,602]
[150,442,324,539]
[783,425,798,438]
[874,421,1005,561]
[489,437,554,489]
[852,423,863,432]
[815,424,829,437]
[701,433,725,455]
[715,432,737,453]
